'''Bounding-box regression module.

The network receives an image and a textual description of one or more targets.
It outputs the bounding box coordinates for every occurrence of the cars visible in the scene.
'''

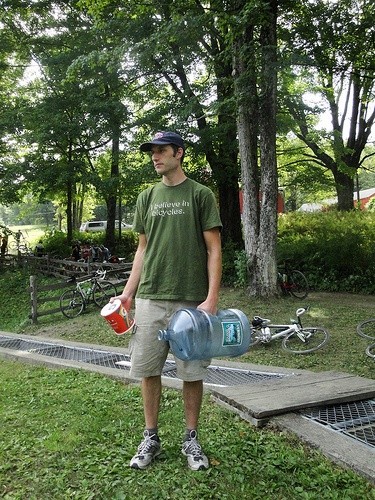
[81,220,133,232]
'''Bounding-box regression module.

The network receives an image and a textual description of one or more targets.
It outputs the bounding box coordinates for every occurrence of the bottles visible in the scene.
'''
[264,327,271,337]
[156,308,250,361]
[86,288,91,300]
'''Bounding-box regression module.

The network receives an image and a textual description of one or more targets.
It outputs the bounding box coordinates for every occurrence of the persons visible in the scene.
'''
[72,240,80,260]
[15,230,22,242]
[81,242,109,259]
[35,240,44,257]
[109,131,222,471]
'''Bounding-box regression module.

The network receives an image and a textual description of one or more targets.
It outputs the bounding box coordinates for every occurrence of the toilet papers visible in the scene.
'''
[100,298,136,335]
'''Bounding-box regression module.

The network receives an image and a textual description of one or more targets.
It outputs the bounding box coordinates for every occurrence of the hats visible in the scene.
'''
[140,131,184,152]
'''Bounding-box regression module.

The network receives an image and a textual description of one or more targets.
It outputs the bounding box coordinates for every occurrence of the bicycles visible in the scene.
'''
[59,270,117,318]
[8,239,28,254]
[277,257,309,300]
[357,318,375,358]
[248,305,329,354]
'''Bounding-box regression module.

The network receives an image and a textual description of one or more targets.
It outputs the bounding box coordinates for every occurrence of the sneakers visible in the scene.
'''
[130,430,161,469]
[182,429,209,471]
